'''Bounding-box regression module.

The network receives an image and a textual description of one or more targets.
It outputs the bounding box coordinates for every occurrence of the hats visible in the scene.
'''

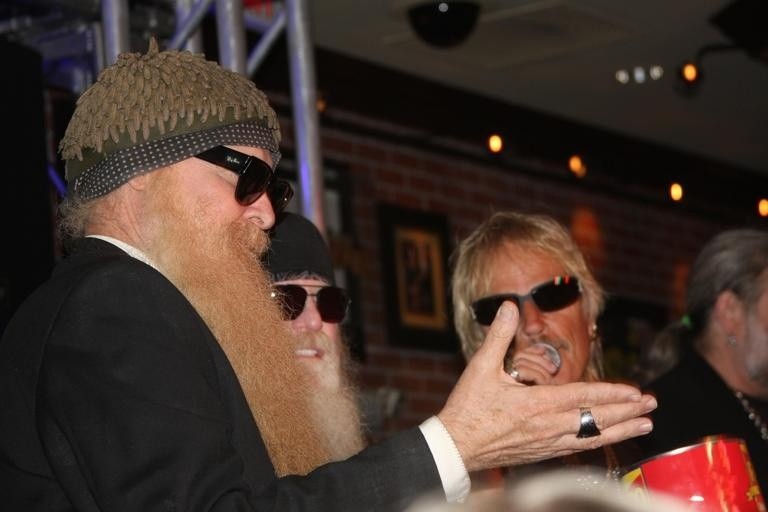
[258,212,337,287]
[60,50,281,180]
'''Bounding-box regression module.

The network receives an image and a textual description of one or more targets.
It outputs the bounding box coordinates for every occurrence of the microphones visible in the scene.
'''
[533,343,561,370]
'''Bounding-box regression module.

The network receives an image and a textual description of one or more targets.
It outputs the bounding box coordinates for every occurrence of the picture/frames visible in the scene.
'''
[379,204,458,352]
[274,147,366,361]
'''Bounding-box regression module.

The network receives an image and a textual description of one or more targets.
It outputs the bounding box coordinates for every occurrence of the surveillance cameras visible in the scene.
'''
[407,3,483,49]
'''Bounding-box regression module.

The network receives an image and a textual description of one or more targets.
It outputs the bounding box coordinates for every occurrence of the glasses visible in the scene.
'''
[194,144,292,216]
[268,284,350,325]
[472,275,582,327]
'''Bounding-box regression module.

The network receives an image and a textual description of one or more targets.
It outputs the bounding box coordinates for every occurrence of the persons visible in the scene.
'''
[450,210,662,512]
[3,48,663,511]
[624,230,767,512]
[257,211,343,394]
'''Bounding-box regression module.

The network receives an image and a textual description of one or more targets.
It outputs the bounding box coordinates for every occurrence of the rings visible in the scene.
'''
[508,369,520,382]
[576,408,600,437]
